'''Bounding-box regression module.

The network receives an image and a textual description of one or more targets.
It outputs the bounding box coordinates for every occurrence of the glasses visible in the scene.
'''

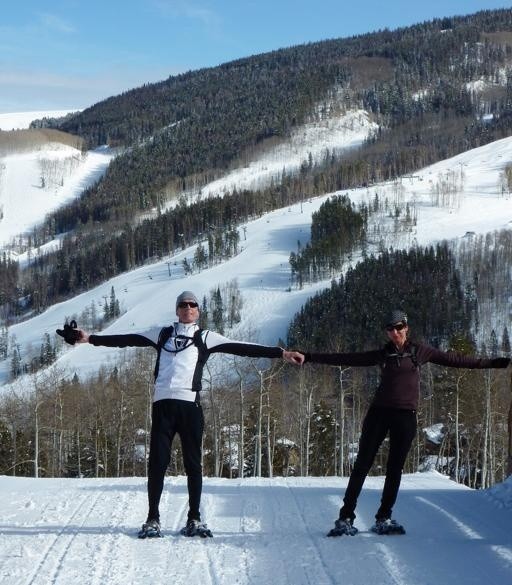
[386,323,405,332]
[178,301,198,308]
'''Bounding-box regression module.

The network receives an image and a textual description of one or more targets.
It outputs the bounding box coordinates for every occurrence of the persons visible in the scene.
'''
[292,308,511,538]
[73,290,306,538]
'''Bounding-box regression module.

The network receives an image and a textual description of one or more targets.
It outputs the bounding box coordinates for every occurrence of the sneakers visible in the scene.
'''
[186,520,200,528]
[376,517,391,525]
[334,517,353,529]
[141,519,161,532]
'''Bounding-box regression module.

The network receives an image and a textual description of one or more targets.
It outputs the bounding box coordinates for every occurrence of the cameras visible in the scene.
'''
[56,320,80,345]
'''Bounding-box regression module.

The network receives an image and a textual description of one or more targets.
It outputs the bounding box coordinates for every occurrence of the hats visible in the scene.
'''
[176,292,199,308]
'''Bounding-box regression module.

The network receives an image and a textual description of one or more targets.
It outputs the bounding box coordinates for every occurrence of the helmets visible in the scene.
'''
[381,310,408,328]
